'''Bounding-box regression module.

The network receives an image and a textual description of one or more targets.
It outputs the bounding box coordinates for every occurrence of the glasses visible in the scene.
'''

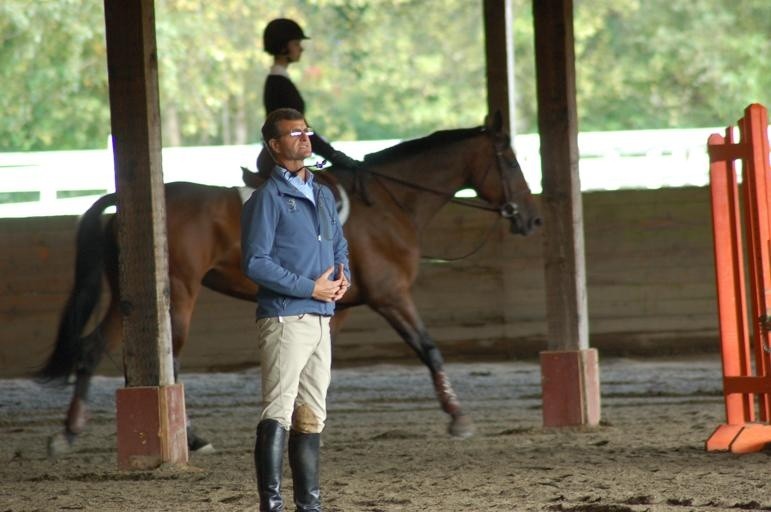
[275,125,315,144]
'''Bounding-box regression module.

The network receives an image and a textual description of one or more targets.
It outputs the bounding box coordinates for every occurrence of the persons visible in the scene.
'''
[253,17,366,176]
[241,107,353,511]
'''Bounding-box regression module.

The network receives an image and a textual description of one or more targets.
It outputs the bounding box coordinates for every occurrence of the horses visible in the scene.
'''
[23,107,544,459]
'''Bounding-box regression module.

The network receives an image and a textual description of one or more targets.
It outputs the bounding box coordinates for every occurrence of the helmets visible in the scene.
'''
[261,18,311,52]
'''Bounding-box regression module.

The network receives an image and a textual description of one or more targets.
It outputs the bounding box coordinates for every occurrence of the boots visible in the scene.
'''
[253,416,285,510]
[287,423,325,512]
